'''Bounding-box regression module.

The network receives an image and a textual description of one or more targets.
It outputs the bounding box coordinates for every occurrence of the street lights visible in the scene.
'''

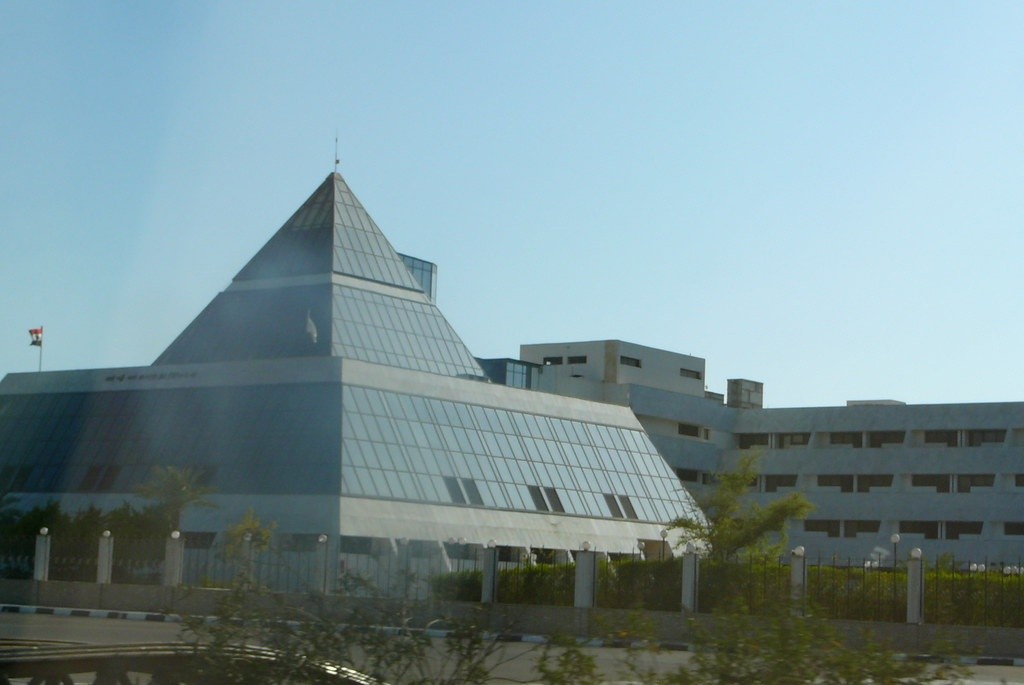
[891,532,902,623]
[660,528,668,560]
[637,541,645,561]
[455,537,469,573]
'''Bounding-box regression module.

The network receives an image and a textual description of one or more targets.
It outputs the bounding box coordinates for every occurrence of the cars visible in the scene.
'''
[0,634,389,685]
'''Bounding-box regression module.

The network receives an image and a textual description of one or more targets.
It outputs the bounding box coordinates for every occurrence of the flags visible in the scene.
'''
[29,328,42,348]
